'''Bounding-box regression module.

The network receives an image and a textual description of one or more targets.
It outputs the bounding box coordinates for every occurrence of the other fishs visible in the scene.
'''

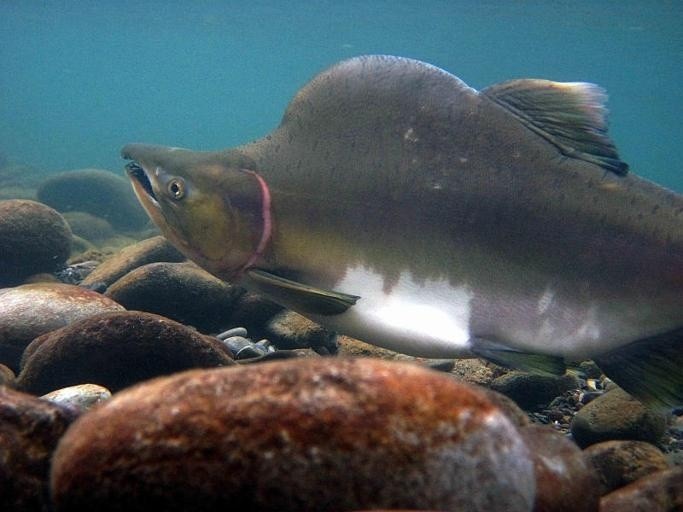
[119,54,683,414]
